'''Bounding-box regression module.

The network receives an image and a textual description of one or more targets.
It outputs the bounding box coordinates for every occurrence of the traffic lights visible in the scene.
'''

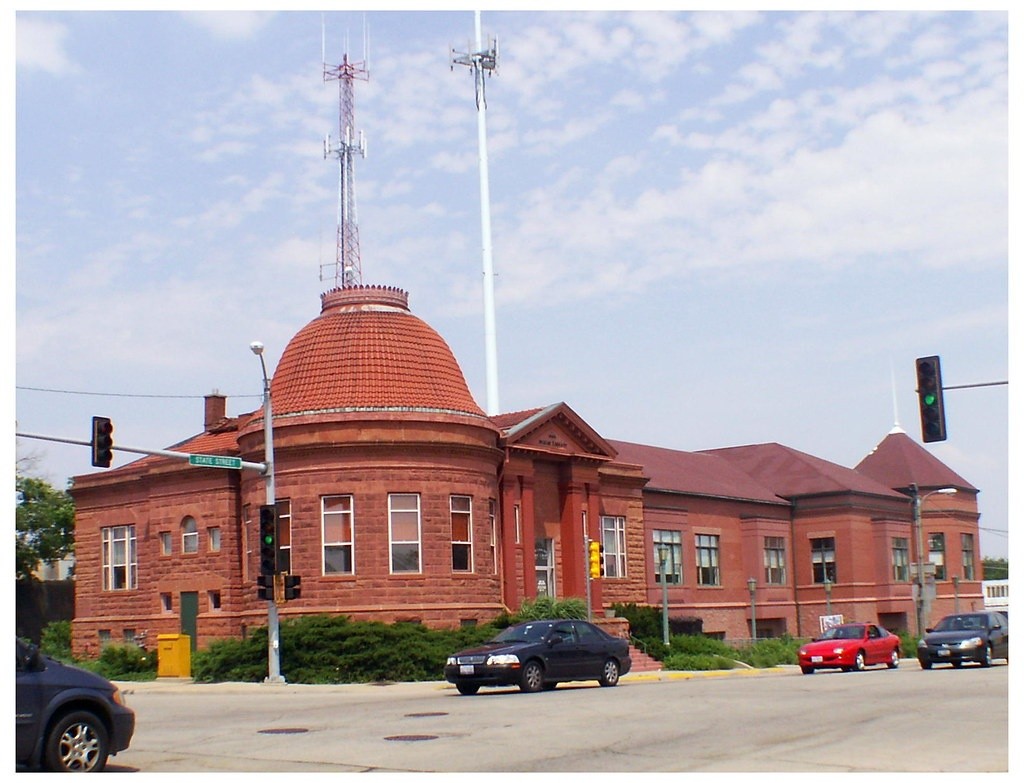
[915,355,947,444]
[90,415,114,469]
[587,541,605,580]
[256,574,275,601]
[258,505,281,575]
[282,575,302,600]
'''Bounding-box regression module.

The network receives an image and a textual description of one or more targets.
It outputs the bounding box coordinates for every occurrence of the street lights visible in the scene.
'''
[907,481,959,640]
[747,577,758,645]
[250,340,286,685]
[951,575,961,616]
[822,578,833,615]
[656,542,672,646]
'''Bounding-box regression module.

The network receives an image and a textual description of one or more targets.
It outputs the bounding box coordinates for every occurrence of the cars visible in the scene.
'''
[444,618,633,696]
[916,610,1008,670]
[797,621,902,674]
[16,634,137,773]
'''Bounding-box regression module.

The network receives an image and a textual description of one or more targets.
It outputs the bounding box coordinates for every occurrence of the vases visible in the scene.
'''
[604,610,616,617]
